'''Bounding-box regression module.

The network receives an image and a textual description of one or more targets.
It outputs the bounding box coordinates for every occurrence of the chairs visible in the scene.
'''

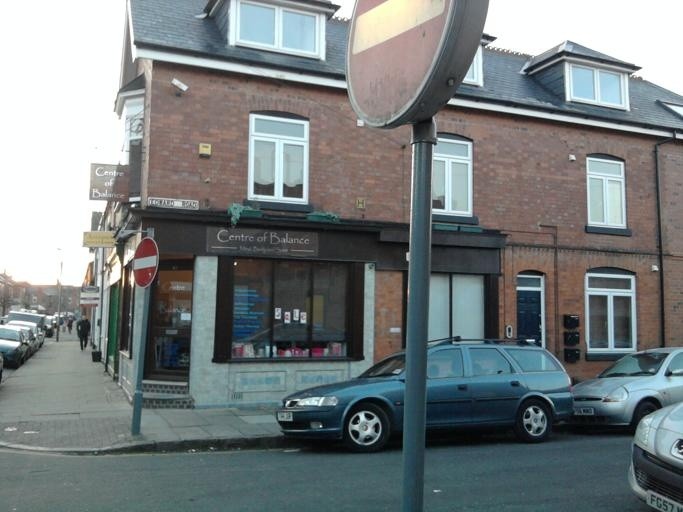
[428,359,489,378]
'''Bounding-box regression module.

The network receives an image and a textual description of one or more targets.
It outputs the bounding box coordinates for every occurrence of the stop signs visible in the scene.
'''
[348,0,488,128]
[133,237,159,287]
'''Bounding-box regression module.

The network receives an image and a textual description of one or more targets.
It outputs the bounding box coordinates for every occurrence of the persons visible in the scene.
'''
[77,316,90,351]
[60,318,73,334]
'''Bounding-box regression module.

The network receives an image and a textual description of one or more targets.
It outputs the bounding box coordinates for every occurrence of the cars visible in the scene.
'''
[1,311,64,368]
[570,347,683,512]
[276,336,574,452]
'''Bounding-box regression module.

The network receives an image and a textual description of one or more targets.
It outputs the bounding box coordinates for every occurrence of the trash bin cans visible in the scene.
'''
[91,351,102,362]
